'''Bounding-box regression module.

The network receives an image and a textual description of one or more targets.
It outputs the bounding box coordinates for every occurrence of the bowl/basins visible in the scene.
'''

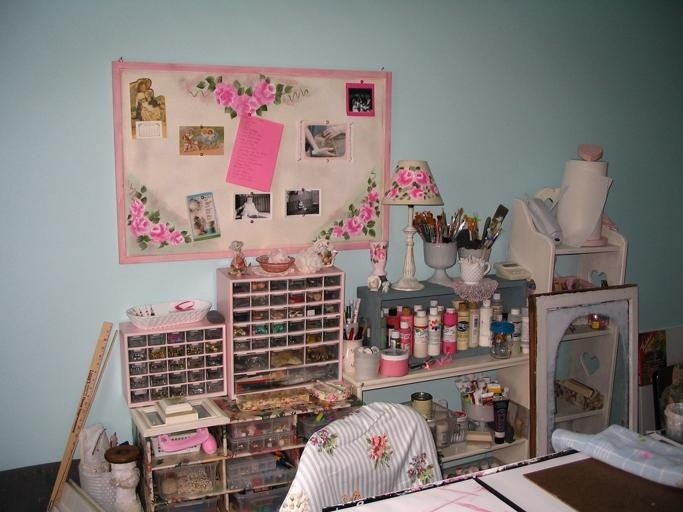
[256,255,295,273]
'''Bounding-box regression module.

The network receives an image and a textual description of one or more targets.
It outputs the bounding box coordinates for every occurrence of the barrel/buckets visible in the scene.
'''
[662,402,683,441]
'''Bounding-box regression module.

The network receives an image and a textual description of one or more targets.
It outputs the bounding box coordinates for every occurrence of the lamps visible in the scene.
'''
[380,159,444,292]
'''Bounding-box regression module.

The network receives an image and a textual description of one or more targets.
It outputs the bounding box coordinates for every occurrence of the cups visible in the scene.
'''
[369,239,388,277]
[459,256,491,285]
[351,344,382,381]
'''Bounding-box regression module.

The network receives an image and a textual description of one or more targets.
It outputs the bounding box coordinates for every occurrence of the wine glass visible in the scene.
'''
[423,240,457,288]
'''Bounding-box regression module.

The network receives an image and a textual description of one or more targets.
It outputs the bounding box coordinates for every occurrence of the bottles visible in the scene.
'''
[379,292,530,359]
[435,410,469,448]
[380,348,409,378]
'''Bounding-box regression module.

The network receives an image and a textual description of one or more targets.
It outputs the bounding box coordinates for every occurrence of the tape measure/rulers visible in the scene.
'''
[44,320,113,511]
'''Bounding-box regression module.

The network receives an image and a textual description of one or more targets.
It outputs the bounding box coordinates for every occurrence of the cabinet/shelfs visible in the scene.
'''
[345,352,529,487]
[131,388,356,510]
[357,280,526,369]
[122,310,227,407]
[508,197,628,462]
[217,268,345,383]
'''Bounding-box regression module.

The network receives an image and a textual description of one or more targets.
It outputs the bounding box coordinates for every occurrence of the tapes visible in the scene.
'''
[381,348,410,361]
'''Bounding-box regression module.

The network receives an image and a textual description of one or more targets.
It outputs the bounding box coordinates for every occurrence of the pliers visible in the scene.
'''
[270,451,294,468]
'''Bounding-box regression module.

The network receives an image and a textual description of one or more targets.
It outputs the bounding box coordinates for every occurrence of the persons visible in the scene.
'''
[303,126,340,158]
[193,216,206,236]
[181,127,218,152]
[295,201,308,211]
[320,124,346,141]
[143,90,160,107]
[238,194,261,218]
[133,80,160,121]
[350,97,370,113]
[205,221,216,236]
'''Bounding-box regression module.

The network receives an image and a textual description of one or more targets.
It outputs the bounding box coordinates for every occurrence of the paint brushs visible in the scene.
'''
[413,205,509,249]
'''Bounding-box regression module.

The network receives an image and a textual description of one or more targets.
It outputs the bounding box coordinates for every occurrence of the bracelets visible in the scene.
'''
[308,149,313,157]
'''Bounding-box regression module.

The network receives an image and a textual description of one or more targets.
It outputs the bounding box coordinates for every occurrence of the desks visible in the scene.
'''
[320,427,683,512]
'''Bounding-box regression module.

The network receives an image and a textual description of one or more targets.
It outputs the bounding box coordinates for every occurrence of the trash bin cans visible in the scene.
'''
[664,403,683,444]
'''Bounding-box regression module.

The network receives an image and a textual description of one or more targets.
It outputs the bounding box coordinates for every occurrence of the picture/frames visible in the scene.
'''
[113,60,393,267]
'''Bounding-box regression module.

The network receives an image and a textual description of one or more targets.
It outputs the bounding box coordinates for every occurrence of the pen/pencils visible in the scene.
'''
[343,297,374,346]
